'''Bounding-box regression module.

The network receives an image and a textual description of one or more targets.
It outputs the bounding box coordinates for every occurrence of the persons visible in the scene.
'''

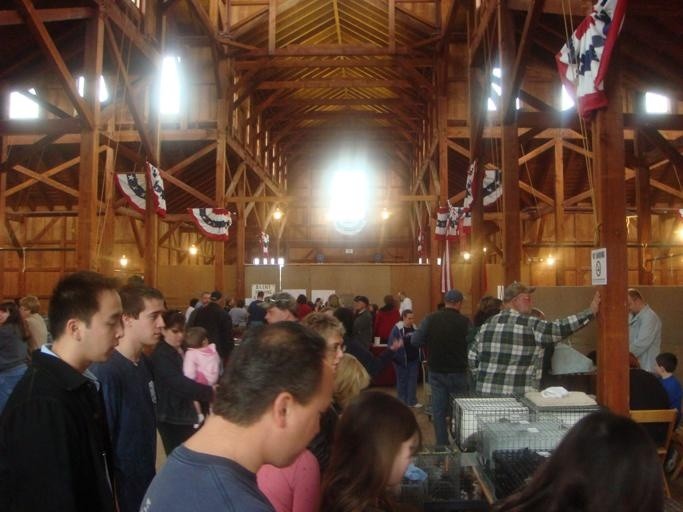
[1,271,683,512]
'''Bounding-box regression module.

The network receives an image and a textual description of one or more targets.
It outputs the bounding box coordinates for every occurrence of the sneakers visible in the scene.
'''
[406,401,425,409]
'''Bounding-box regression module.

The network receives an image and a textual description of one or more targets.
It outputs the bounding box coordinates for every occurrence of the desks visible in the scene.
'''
[366,344,399,388]
[222,328,246,369]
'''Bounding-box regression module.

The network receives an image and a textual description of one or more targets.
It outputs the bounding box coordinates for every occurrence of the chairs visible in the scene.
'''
[670,430,683,484]
[628,408,679,500]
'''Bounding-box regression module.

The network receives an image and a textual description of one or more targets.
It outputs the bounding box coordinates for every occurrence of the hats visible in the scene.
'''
[501,280,538,302]
[209,290,222,301]
[255,289,299,311]
[443,288,464,303]
